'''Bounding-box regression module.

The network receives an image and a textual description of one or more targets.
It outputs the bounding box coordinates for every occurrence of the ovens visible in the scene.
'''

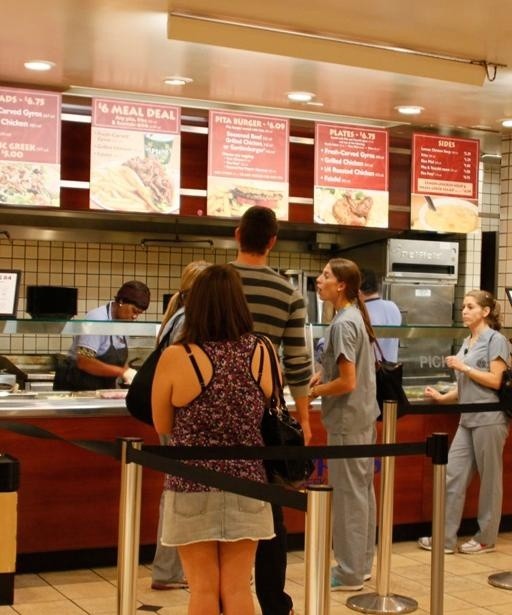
[339,238,459,385]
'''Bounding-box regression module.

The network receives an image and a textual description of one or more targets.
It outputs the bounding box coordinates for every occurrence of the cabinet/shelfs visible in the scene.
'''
[0,320,512,554]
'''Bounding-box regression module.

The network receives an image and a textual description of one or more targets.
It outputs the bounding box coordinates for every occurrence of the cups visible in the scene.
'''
[143,132,175,165]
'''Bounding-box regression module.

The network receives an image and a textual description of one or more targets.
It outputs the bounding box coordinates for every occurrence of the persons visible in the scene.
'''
[151,260,214,589]
[417,290,510,554]
[227,206,312,614]
[355,269,402,365]
[53,281,150,391]
[151,267,282,615]
[307,258,382,592]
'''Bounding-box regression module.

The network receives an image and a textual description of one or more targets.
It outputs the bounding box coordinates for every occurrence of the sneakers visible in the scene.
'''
[331,577,363,590]
[418,537,455,553]
[458,538,496,554]
[364,574,372,582]
[152,581,190,590]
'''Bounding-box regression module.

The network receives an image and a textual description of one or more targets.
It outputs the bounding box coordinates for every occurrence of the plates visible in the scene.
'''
[91,160,179,213]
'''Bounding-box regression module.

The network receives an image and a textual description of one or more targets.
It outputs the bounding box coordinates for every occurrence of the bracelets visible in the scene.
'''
[466,366,472,373]
[309,384,317,397]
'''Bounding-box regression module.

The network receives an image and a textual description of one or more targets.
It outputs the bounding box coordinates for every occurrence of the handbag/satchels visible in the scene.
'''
[125,348,163,426]
[374,359,410,422]
[262,405,314,489]
[496,370,512,421]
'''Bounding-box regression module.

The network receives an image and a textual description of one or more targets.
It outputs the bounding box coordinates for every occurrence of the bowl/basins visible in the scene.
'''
[420,197,478,234]
[234,188,284,208]
